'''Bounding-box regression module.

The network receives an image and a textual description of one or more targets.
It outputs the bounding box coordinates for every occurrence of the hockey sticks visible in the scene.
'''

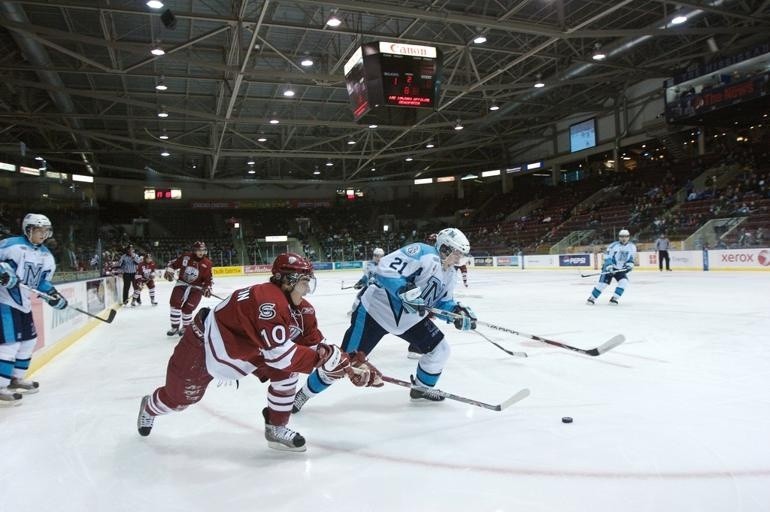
[20,282,116,323]
[424,307,625,356]
[341,281,357,289]
[577,267,628,278]
[351,367,531,412]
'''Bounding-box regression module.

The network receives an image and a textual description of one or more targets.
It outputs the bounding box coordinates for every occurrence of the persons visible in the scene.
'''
[137,252,383,449]
[291,227,476,416]
[0,213,67,401]
[164,240,212,336]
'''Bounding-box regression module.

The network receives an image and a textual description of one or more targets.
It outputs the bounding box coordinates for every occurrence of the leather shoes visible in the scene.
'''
[587,297,595,303]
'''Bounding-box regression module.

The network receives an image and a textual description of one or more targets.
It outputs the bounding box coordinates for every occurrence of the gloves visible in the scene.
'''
[454,302,475,330]
[316,342,349,380]
[49,292,67,310]
[353,280,363,290]
[202,285,213,296]
[0,261,18,289]
[397,284,428,316]
[624,261,634,271]
[165,268,176,280]
[351,350,383,388]
[606,265,617,276]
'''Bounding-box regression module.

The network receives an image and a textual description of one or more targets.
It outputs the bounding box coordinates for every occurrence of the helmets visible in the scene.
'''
[190,241,208,263]
[427,226,472,271]
[143,254,153,264]
[618,229,632,238]
[373,247,386,257]
[270,252,318,308]
[21,212,54,239]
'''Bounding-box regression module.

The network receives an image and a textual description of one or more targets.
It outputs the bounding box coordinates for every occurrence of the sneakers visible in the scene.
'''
[264,422,306,448]
[291,388,311,415]
[0,386,23,401]
[609,297,618,304]
[137,394,156,437]
[166,327,187,338]
[409,374,444,401]
[464,280,468,287]
[7,378,40,390]
[120,300,158,307]
